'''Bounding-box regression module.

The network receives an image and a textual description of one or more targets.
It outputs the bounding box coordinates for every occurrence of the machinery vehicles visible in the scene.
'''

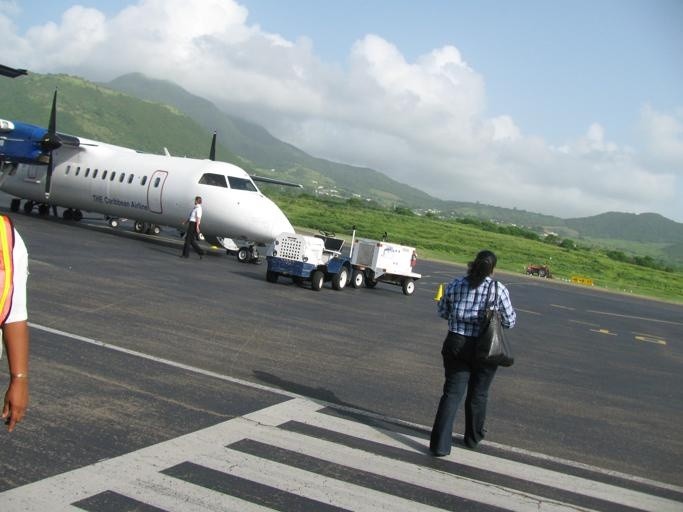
[525,259,548,278]
[266,230,353,292]
[348,237,423,297]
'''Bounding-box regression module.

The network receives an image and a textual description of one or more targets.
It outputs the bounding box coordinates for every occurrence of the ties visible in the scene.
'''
[187,208,195,221]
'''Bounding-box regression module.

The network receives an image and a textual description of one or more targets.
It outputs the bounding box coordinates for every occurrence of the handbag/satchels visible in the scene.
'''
[475,281,514,368]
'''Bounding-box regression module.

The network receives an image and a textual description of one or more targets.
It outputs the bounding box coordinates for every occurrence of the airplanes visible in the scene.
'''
[0,62,304,264]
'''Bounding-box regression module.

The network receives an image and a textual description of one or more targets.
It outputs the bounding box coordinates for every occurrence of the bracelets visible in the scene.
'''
[11,373,28,378]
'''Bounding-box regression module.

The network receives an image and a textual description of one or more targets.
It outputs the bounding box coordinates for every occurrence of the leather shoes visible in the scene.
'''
[180,255,189,258]
[200,252,206,259]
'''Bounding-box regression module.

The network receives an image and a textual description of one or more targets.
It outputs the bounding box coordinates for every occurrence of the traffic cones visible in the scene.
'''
[433,283,444,301]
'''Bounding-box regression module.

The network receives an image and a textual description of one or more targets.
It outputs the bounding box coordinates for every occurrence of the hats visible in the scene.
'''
[196,233,205,241]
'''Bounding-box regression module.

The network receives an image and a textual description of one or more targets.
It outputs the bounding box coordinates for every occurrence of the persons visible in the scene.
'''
[430,250,517,456]
[0,212,29,433]
[179,196,205,260]
